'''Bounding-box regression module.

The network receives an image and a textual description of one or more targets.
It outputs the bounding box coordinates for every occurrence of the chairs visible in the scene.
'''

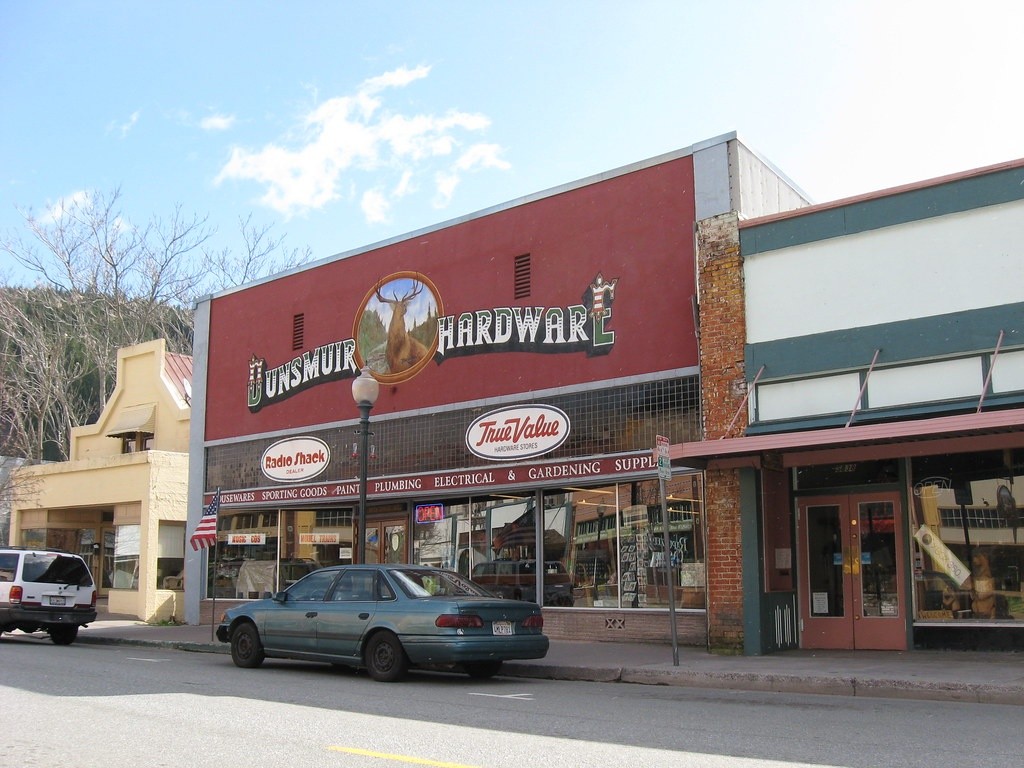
[334,582,352,601]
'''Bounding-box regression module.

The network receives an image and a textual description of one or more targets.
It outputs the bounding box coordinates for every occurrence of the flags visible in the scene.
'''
[189,491,219,552]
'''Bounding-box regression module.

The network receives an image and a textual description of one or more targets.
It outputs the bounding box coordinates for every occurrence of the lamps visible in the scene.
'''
[92,542,100,556]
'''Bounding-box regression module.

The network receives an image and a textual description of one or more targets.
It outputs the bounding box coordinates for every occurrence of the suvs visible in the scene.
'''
[1,545,98,646]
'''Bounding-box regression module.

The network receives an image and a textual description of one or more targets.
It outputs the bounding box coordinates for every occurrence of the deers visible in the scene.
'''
[373,270,429,375]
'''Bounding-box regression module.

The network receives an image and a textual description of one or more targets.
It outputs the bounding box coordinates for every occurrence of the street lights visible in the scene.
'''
[351,364,380,564]
[594,499,605,586]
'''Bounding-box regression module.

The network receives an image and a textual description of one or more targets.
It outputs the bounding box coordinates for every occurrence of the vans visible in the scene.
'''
[235,556,333,602]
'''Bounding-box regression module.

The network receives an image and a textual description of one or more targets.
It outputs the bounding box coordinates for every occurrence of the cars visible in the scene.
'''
[466,559,574,606]
[860,570,972,619]
[216,565,548,682]
